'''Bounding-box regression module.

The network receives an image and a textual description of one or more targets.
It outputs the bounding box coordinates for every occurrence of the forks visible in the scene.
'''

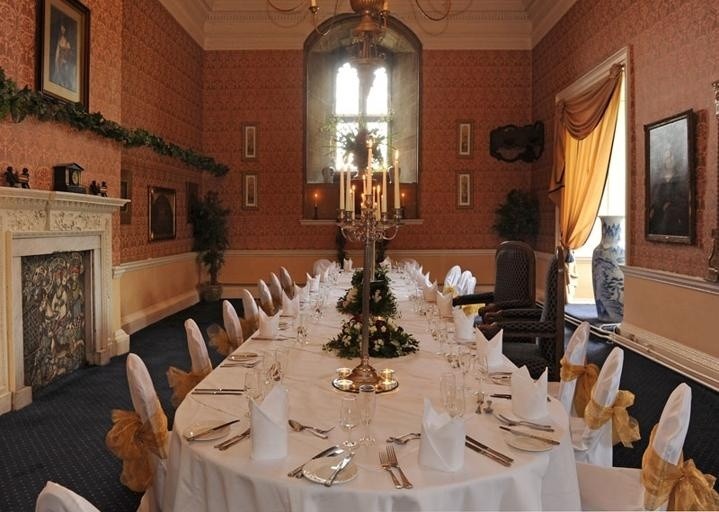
[214,426,251,449]
[302,424,337,433]
[475,392,484,416]
[386,445,414,488]
[379,451,402,488]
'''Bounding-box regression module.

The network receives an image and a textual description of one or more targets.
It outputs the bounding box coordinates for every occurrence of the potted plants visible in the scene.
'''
[189,190,230,303]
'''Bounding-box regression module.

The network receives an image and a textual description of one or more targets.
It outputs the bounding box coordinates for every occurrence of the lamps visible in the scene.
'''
[265,0,451,59]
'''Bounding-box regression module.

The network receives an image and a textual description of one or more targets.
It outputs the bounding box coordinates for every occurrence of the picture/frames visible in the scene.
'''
[242,123,259,161]
[455,170,474,209]
[36,0,91,115]
[148,186,176,242]
[456,119,474,159]
[242,172,260,210]
[644,108,696,246]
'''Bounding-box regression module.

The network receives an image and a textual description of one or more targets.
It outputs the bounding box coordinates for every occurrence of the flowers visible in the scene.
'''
[314,110,400,170]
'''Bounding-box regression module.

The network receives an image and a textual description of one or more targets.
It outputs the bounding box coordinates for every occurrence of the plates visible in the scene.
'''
[503,430,555,452]
[184,418,232,442]
[303,457,358,486]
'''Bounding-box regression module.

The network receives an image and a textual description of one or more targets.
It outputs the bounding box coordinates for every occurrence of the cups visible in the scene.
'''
[453,371,466,417]
[440,372,458,409]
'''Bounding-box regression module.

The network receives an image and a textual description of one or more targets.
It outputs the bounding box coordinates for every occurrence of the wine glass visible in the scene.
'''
[257,369,273,400]
[358,385,377,445]
[337,394,359,450]
[469,354,489,399]
[241,369,262,418]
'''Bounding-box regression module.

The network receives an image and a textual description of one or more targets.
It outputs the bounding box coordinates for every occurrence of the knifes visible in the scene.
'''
[194,387,243,392]
[192,390,243,395]
[499,414,551,428]
[466,441,511,467]
[323,451,355,487]
[186,419,240,441]
[464,434,515,461]
[492,414,555,433]
[295,449,344,479]
[218,432,249,451]
[386,431,420,442]
[499,425,561,446]
[287,445,336,478]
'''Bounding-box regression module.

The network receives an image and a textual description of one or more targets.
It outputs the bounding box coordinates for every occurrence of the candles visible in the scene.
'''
[315,194,318,207]
[340,139,405,221]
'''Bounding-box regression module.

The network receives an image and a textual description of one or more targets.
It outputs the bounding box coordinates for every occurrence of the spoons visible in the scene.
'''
[482,399,494,416]
[390,436,420,445]
[288,419,328,439]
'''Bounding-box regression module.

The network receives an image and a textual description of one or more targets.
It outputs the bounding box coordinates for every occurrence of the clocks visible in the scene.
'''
[53,162,85,191]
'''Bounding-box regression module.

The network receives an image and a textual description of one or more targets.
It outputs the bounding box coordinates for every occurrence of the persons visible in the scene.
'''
[650,150,689,236]
[52,24,73,91]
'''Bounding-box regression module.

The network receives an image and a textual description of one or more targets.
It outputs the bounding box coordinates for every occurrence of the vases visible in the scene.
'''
[592,215,626,323]
